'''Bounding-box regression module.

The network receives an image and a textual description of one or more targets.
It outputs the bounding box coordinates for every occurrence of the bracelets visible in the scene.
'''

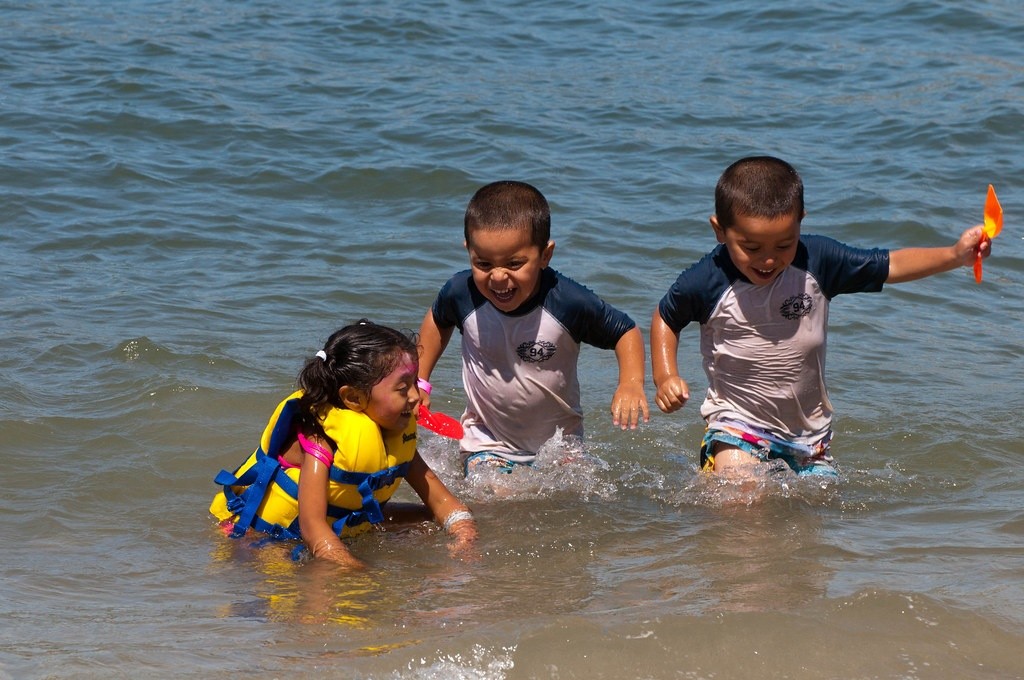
[312,536,349,559]
[417,378,432,394]
[444,511,476,533]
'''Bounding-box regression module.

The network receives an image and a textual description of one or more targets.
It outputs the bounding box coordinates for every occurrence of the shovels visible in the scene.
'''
[973,184,1004,283]
[416,405,464,439]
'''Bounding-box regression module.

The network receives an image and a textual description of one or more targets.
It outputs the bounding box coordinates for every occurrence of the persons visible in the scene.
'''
[208,318,477,564]
[416,180,650,495]
[651,156,993,480]
